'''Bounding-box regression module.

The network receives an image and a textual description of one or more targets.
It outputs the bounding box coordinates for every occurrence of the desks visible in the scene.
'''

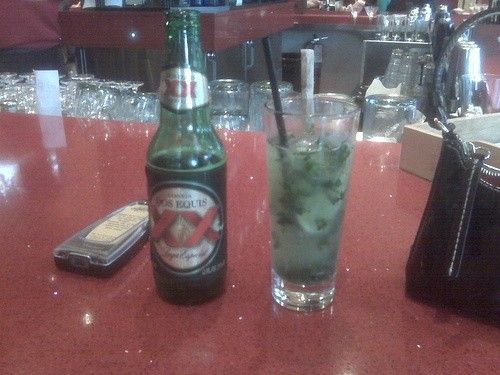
[282,7,500,112]
[58,0,295,148]
[0,112,500,375]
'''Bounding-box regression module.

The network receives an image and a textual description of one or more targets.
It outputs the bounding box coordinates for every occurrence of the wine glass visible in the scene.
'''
[348,3,364,25]
[363,5,379,25]
[0,72,159,121]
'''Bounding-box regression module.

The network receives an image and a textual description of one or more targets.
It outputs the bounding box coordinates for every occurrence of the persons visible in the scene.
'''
[0,0,63,75]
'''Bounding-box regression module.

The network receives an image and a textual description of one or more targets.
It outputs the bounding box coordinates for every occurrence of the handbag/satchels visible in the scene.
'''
[405,7,500,322]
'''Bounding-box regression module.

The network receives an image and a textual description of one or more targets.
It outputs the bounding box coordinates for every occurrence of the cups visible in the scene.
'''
[264,98,359,311]
[362,94,417,142]
[248,81,293,129]
[390,14,408,40]
[207,78,250,131]
[374,14,392,40]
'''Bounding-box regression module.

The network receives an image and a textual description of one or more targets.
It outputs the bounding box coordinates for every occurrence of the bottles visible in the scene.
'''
[384,3,488,117]
[146,9,228,304]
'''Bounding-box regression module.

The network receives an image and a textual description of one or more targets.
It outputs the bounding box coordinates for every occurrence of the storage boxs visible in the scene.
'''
[400,113,499,181]
